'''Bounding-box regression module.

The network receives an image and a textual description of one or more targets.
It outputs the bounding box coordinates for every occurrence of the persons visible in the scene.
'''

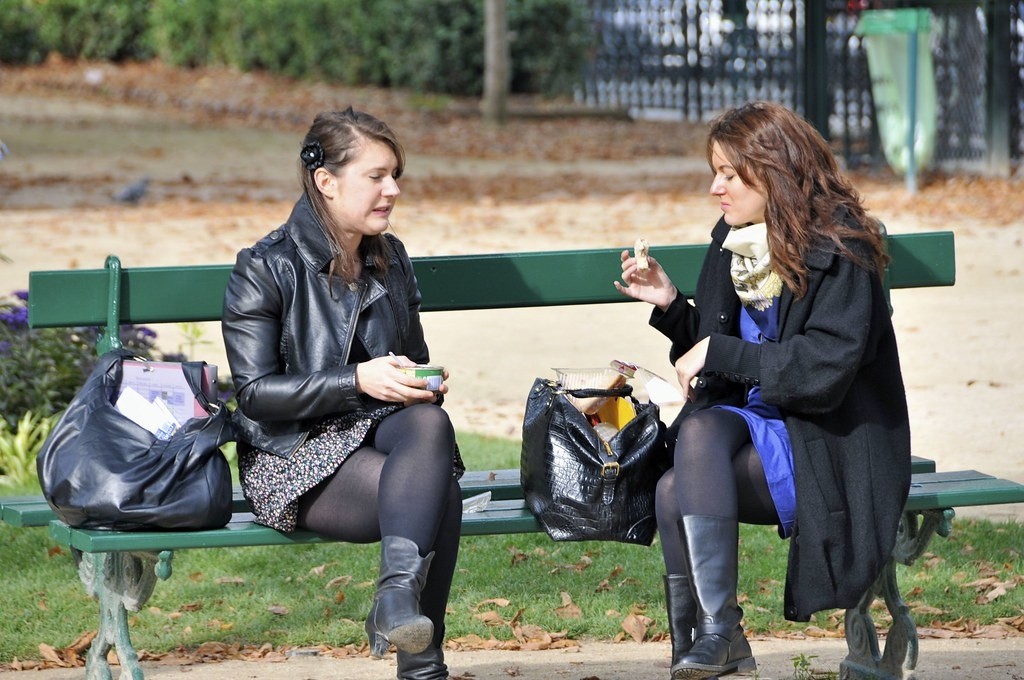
[222,106,465,680]
[614,103,910,680]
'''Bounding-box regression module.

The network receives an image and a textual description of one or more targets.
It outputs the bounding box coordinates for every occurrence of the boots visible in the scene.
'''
[396,621,451,680]
[667,513,758,680]
[661,574,719,680]
[364,535,436,660]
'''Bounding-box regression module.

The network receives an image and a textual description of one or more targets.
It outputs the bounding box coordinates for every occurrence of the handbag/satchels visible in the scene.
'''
[520,376,671,546]
[35,349,235,532]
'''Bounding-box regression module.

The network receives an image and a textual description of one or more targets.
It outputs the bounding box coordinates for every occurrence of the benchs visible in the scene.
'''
[0,229,1023,679]
